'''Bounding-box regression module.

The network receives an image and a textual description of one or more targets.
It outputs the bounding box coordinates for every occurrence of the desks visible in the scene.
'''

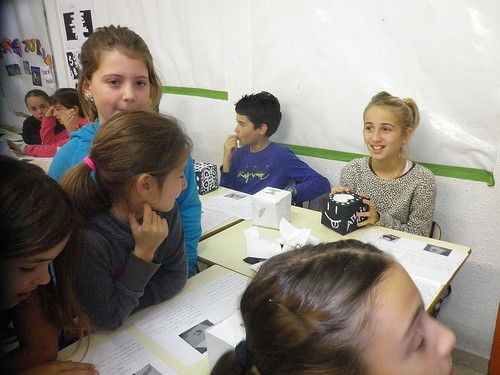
[0,127,472,375]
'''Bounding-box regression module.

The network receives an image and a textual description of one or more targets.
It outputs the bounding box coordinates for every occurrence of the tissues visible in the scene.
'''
[321,189,370,236]
[194,161,219,195]
[251,186,291,229]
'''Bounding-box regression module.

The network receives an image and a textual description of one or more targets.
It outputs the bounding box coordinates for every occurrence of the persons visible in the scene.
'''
[209,238,457,375]
[219,92,331,207]
[329,91,437,238]
[48,24,202,280]
[0,152,100,375]
[44,109,194,344]
[22,89,66,146]
[3,88,94,158]
